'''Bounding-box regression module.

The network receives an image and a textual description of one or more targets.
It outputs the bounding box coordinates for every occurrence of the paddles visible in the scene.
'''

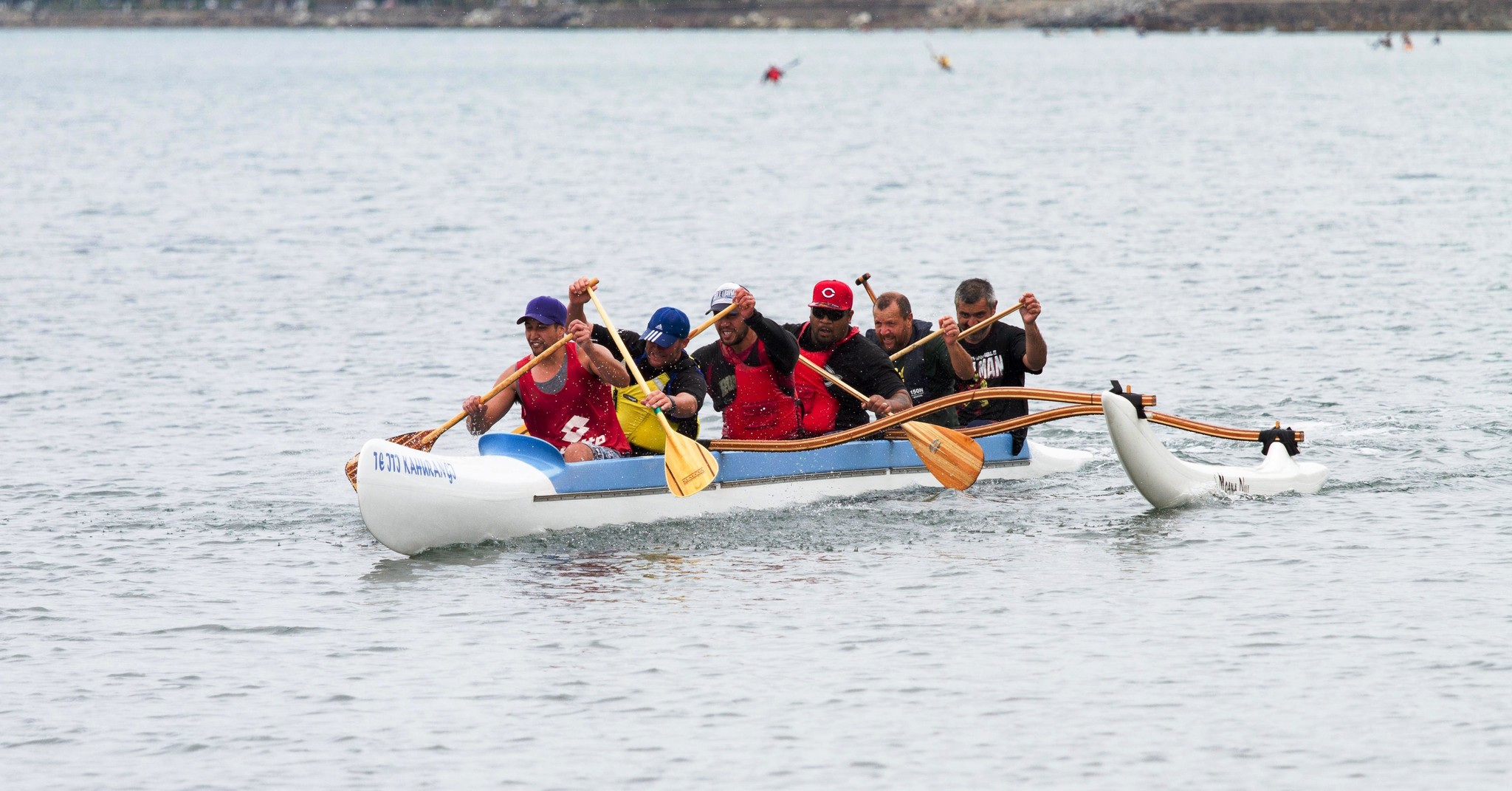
[798,354,985,491]
[585,276,721,499]
[888,319,955,361]
[345,328,580,494]
[688,300,749,340]
[956,301,1027,342]
[855,272,877,304]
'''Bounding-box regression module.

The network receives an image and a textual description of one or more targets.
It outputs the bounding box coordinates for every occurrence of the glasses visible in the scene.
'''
[812,307,850,321]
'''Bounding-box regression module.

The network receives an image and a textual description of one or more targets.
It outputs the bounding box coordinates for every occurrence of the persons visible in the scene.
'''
[865,291,974,431]
[954,278,1047,456]
[781,280,912,438]
[689,282,802,440]
[564,277,706,457]
[462,296,632,462]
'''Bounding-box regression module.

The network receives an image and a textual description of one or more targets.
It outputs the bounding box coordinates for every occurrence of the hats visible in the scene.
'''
[516,296,567,327]
[808,279,853,311]
[638,307,690,348]
[705,282,750,315]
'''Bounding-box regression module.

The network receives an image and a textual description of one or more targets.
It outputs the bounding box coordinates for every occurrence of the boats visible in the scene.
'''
[353,401,1036,560]
